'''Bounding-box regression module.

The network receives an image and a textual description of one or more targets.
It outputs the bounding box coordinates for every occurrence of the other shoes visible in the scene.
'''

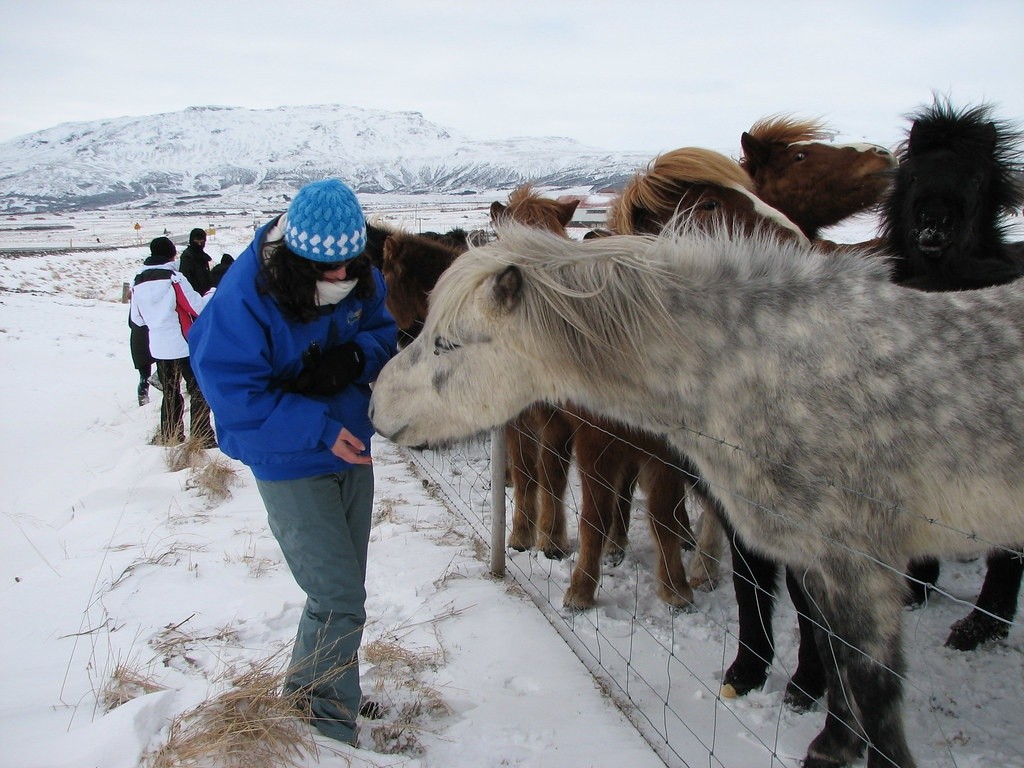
[157,435,186,446]
[295,699,387,749]
[192,437,218,449]
[139,387,148,405]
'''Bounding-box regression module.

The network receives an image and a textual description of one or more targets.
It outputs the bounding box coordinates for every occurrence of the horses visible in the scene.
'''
[364,86,1024,768]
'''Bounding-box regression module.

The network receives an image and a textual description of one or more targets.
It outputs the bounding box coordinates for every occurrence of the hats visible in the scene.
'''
[220,254,233,264]
[145,237,178,264]
[284,179,369,262]
[190,229,204,240]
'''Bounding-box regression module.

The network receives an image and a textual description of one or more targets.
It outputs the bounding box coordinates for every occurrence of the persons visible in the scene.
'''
[187,177,398,750]
[131,237,217,449]
[210,253,234,288]
[179,228,212,321]
[128,307,163,407]
